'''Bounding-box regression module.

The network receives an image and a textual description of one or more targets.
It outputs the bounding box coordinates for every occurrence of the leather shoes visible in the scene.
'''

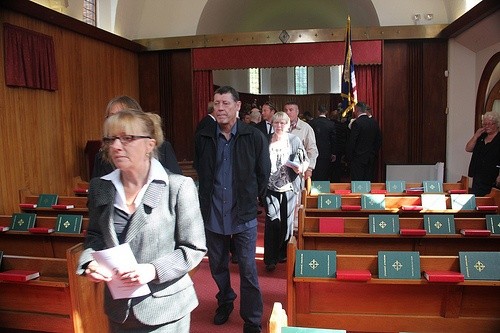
[213,311,229,325]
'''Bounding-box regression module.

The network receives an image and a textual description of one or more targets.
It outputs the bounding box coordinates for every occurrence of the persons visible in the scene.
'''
[465,112,500,197]
[193,85,272,333]
[73,109,208,333]
[193,100,383,273]
[86,94,182,210]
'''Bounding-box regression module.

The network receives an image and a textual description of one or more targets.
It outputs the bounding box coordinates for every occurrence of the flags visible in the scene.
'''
[341,23,358,130]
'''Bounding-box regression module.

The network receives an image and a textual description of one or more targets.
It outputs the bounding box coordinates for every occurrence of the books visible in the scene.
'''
[0,270,40,283]
[294,249,336,277]
[377,250,421,279]
[75,183,89,193]
[52,205,74,209]
[9,213,37,232]
[310,179,469,196]
[459,251,500,281]
[17,203,37,208]
[55,215,83,234]
[37,194,59,208]
[317,193,500,211]
[336,270,371,280]
[317,215,500,237]
[0,226,10,231]
[422,269,464,282]
[29,227,56,233]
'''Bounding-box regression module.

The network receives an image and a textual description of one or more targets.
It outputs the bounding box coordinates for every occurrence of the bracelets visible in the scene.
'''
[307,169,313,172]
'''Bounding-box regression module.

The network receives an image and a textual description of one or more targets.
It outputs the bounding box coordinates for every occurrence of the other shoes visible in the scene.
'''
[278,254,286,262]
[232,254,239,264]
[266,265,275,272]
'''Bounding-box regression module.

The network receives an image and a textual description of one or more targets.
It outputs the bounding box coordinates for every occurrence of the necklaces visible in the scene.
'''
[125,188,141,207]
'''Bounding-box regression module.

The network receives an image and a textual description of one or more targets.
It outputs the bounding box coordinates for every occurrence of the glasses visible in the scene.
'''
[103,135,152,146]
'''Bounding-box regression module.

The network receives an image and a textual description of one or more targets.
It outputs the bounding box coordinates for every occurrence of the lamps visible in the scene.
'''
[414,14,433,25]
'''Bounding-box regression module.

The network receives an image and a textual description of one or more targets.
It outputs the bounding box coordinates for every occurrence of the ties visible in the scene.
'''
[267,122,273,135]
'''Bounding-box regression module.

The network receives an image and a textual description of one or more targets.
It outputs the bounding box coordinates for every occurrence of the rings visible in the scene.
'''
[90,266,96,272]
[129,273,134,279]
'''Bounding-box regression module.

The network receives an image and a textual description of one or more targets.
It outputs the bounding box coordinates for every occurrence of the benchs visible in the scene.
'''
[0,181,112,333]
[288,174,500,333]
